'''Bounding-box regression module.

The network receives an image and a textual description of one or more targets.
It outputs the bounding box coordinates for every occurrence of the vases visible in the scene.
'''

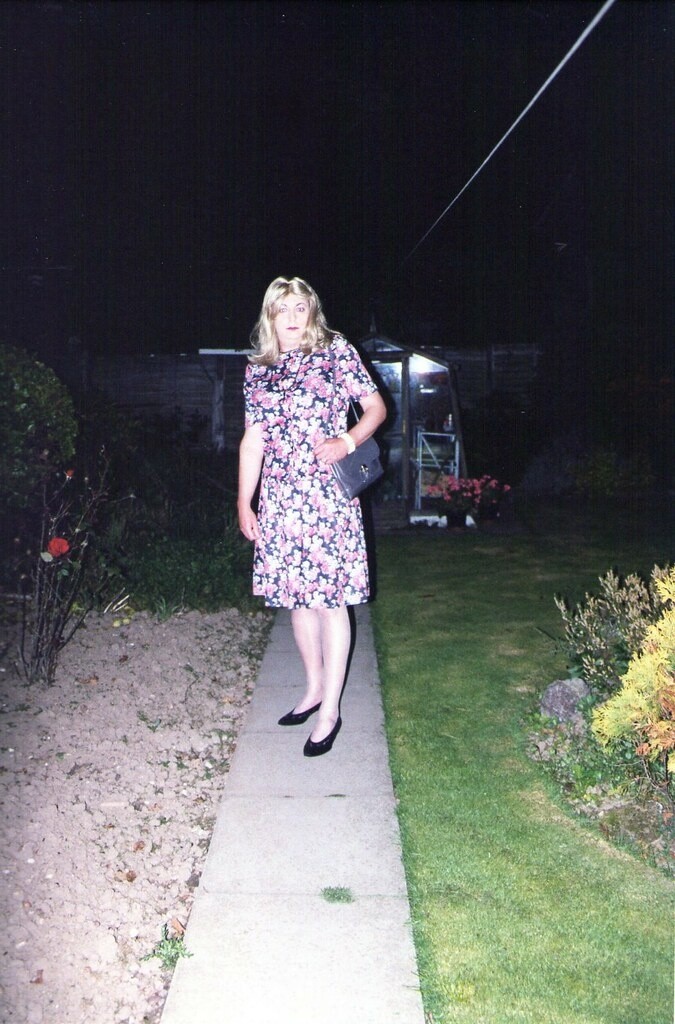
[479,503,498,519]
[437,511,465,527]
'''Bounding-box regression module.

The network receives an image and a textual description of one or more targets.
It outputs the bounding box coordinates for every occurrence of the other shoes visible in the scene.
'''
[277,700,322,725]
[303,716,342,756]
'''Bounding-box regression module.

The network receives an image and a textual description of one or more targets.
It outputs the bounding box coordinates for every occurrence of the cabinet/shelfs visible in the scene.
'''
[414,426,460,512]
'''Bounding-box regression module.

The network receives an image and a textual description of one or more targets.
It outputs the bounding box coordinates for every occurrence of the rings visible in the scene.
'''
[322,458,326,462]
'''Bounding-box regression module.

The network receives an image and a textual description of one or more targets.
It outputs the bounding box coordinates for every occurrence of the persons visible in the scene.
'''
[236,276,387,758]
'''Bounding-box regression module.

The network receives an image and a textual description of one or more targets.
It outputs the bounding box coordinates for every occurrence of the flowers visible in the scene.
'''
[421,473,510,507]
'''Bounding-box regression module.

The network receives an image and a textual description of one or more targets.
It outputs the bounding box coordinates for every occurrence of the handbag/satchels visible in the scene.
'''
[329,345,385,501]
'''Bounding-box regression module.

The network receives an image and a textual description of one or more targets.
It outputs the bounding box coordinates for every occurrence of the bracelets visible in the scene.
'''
[339,433,356,453]
